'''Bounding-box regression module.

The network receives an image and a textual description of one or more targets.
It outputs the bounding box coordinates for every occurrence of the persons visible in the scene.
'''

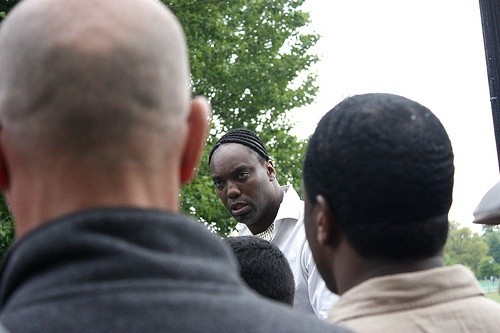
[301,91,500,333]
[218,236,296,310]
[0,0,352,332]
[208,128,344,324]
